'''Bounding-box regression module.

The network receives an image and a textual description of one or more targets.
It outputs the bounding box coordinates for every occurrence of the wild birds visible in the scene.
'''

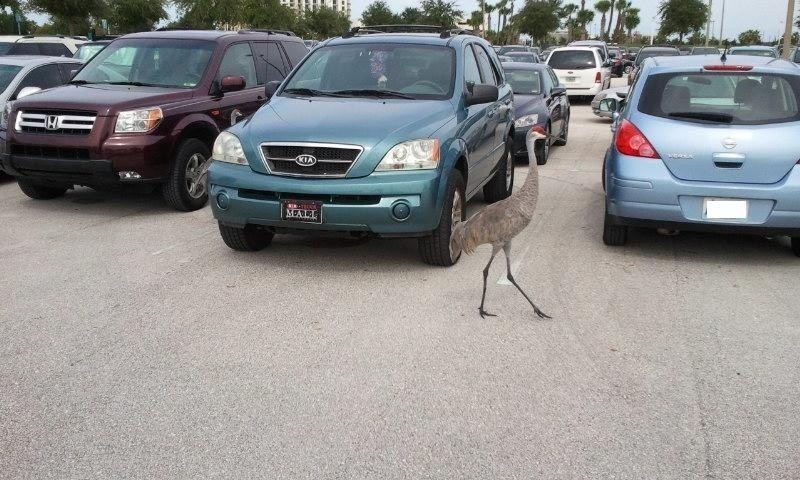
[449,124,567,320]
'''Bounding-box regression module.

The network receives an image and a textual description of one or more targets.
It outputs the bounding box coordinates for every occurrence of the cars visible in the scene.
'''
[501,62,572,164]
[591,75,750,118]
[0,55,129,175]
[602,53,800,249]
[567,38,800,77]
[492,45,565,63]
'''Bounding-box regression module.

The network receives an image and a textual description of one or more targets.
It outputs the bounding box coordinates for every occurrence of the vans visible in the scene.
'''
[547,48,611,98]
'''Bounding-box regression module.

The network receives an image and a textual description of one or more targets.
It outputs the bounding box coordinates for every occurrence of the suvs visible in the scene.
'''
[5,29,313,213]
[0,33,124,60]
[206,26,517,268]
